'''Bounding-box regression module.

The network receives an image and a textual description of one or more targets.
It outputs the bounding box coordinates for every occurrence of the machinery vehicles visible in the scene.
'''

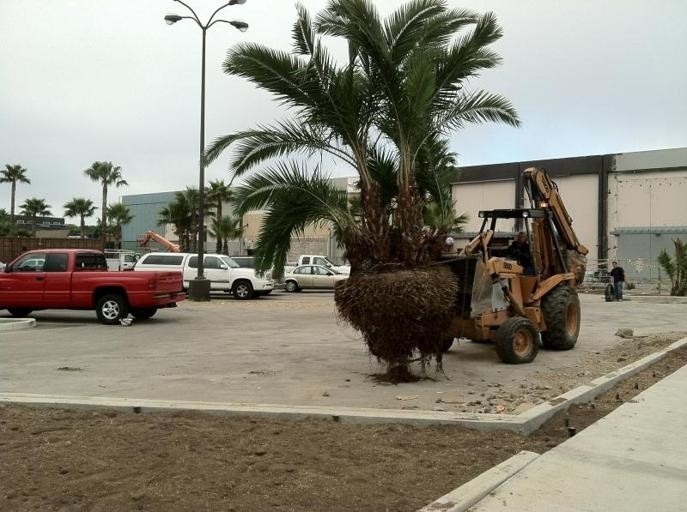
[136,230,180,253]
[441,167,588,364]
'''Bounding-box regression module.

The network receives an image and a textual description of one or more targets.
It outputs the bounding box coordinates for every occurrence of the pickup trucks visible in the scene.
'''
[285,255,351,274]
[0,249,185,325]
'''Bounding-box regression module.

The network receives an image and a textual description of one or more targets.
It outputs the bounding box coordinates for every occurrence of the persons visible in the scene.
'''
[606,261,628,302]
[505,231,525,261]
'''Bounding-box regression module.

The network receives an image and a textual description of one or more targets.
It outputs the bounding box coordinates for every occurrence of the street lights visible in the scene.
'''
[164,0,248,302]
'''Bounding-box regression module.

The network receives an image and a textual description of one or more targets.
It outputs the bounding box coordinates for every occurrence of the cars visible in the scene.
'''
[17,259,45,271]
[284,265,349,292]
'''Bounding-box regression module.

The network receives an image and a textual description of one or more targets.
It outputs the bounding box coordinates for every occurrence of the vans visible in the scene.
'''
[103,249,140,271]
[133,252,274,300]
